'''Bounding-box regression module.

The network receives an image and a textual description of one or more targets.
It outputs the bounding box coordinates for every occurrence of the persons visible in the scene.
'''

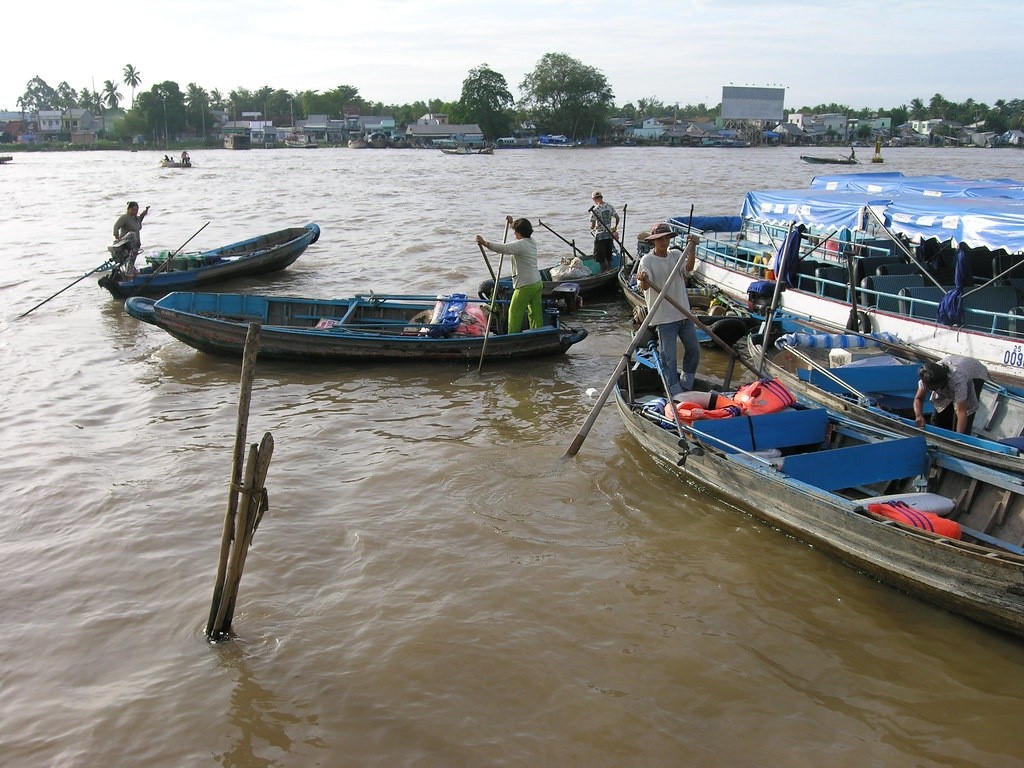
[913,353,989,435]
[112,201,150,274]
[636,224,702,393]
[848,146,855,162]
[590,189,619,273]
[181,151,189,163]
[164,154,175,164]
[474,214,543,335]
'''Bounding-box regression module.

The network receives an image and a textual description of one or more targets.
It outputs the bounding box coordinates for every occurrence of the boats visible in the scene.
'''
[478,248,627,307]
[658,170,1024,397]
[348,138,368,149]
[284,125,318,148]
[872,133,884,163]
[123,292,589,365]
[613,326,1024,640]
[618,255,762,351]
[367,131,388,148]
[440,142,498,154]
[98,227,315,300]
[800,156,857,164]
[388,129,410,148]
[497,138,543,148]
[161,156,191,168]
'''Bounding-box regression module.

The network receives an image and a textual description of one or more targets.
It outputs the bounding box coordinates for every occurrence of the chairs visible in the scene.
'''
[815,267,849,301]
[854,255,907,305]
[860,273,926,313]
[876,261,935,287]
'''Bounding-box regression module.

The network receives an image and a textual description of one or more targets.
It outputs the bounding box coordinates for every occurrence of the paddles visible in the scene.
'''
[588,205,634,260]
[539,219,585,256]
[130,221,211,295]
[839,154,857,163]
[686,204,693,245]
[141,206,151,221]
[756,220,796,381]
[618,204,627,272]
[477,217,510,374]
[478,242,497,282]
[843,250,859,332]
[566,239,693,456]
[641,273,765,379]
[22,257,116,317]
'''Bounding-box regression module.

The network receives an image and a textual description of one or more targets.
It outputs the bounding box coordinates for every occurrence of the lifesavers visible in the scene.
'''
[123,296,158,327]
[666,392,746,427]
[304,223,321,244]
[846,311,872,335]
[753,253,771,276]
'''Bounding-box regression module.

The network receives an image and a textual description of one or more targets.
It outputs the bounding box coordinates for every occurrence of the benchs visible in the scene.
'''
[337,301,359,325]
[809,365,923,395]
[791,262,832,294]
[897,286,1017,337]
[691,407,830,456]
[767,435,928,491]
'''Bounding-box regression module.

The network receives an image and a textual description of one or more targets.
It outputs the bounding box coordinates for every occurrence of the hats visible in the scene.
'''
[592,190,603,199]
[644,223,678,241]
